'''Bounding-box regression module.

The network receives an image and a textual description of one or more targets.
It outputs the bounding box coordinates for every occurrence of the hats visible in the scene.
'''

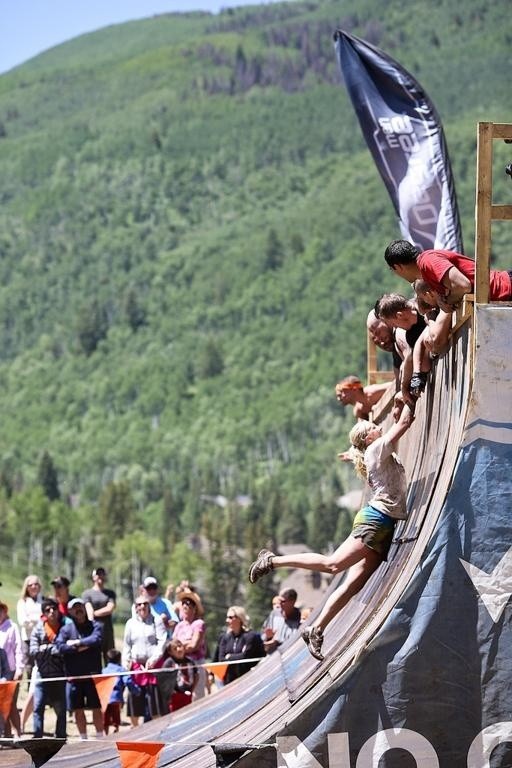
[179,591,204,616]
[143,576,158,589]
[51,576,70,586]
[66,598,85,609]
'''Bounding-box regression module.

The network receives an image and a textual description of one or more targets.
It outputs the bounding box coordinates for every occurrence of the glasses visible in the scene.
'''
[181,600,191,605]
[53,585,61,588]
[226,615,235,619]
[278,598,289,603]
[44,606,57,612]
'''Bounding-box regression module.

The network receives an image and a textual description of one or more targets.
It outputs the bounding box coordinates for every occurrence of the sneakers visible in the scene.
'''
[248,547,276,584]
[301,626,325,661]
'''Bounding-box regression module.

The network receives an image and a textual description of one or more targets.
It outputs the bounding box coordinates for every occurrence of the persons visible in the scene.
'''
[249,391,416,661]
[1,566,314,739]
[335,240,512,420]
